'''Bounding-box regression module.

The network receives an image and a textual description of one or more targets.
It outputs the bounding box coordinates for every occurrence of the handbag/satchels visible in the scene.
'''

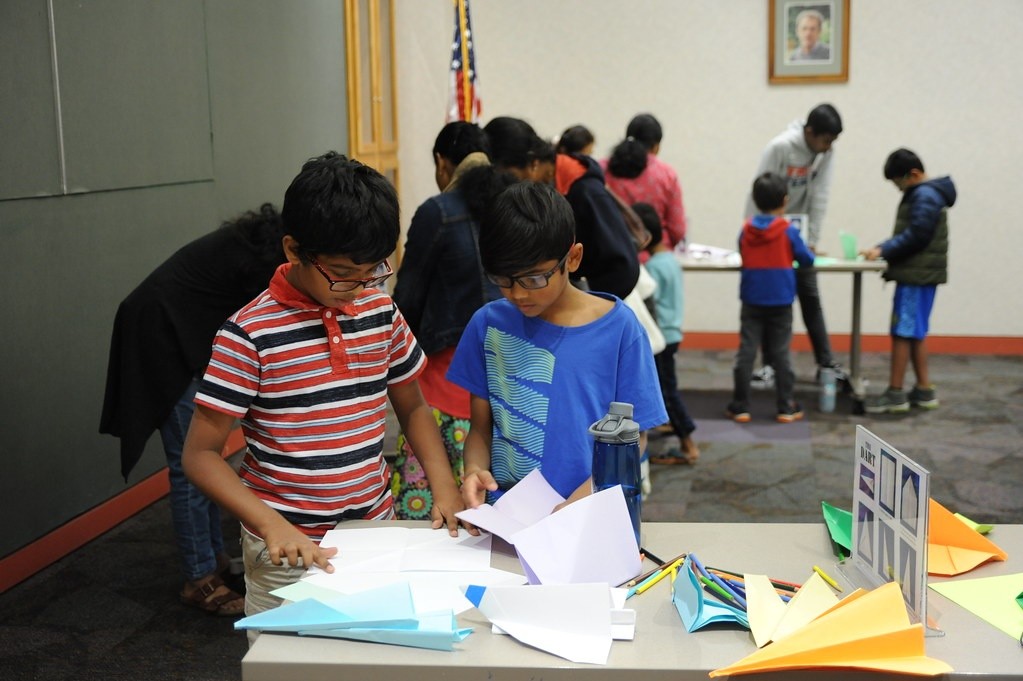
[604,185,649,252]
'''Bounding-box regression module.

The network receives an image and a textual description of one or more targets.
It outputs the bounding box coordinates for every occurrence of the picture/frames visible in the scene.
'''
[768,0,850,83]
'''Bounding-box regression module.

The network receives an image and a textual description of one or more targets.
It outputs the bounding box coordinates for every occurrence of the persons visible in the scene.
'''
[97,202,288,618]
[180,150,480,648]
[725,173,815,422]
[391,111,698,521]
[857,149,957,412]
[443,183,670,533]
[742,105,847,385]
[788,9,829,61]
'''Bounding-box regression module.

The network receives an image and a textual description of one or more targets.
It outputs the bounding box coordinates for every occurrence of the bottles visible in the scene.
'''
[588,402,641,552]
[819,365,836,413]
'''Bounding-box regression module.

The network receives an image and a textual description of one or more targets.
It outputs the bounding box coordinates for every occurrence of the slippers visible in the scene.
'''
[177,574,247,616]
[216,557,248,595]
[650,447,697,465]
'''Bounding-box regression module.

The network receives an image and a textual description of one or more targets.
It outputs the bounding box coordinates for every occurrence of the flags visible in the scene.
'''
[445,0,480,127]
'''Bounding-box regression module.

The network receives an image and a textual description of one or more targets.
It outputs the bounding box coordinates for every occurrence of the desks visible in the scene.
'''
[670,248,893,410]
[241,510,1023,681]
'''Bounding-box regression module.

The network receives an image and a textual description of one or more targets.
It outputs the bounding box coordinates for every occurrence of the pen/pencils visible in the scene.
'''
[728,579,791,602]
[716,575,786,596]
[636,558,685,594]
[700,583,747,613]
[670,568,678,594]
[835,543,845,564]
[700,576,734,601]
[627,553,687,587]
[627,569,663,600]
[769,579,802,588]
[705,567,799,592]
[812,566,843,593]
[678,566,681,571]
[720,578,746,600]
[691,560,698,577]
[711,573,746,608]
[688,551,712,580]
[640,548,666,566]
[707,569,795,597]
[640,553,645,560]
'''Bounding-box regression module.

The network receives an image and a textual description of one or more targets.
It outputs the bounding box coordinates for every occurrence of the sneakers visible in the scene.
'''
[863,388,909,414]
[775,399,805,422]
[724,401,752,422]
[904,384,939,409]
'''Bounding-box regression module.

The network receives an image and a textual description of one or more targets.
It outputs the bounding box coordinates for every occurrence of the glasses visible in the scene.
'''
[482,244,576,290]
[297,246,394,292]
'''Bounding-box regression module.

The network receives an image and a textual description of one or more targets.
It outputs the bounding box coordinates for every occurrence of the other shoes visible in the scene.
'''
[653,422,676,434]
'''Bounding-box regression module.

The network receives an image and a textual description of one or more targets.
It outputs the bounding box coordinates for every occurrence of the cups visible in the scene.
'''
[839,233,857,259]
